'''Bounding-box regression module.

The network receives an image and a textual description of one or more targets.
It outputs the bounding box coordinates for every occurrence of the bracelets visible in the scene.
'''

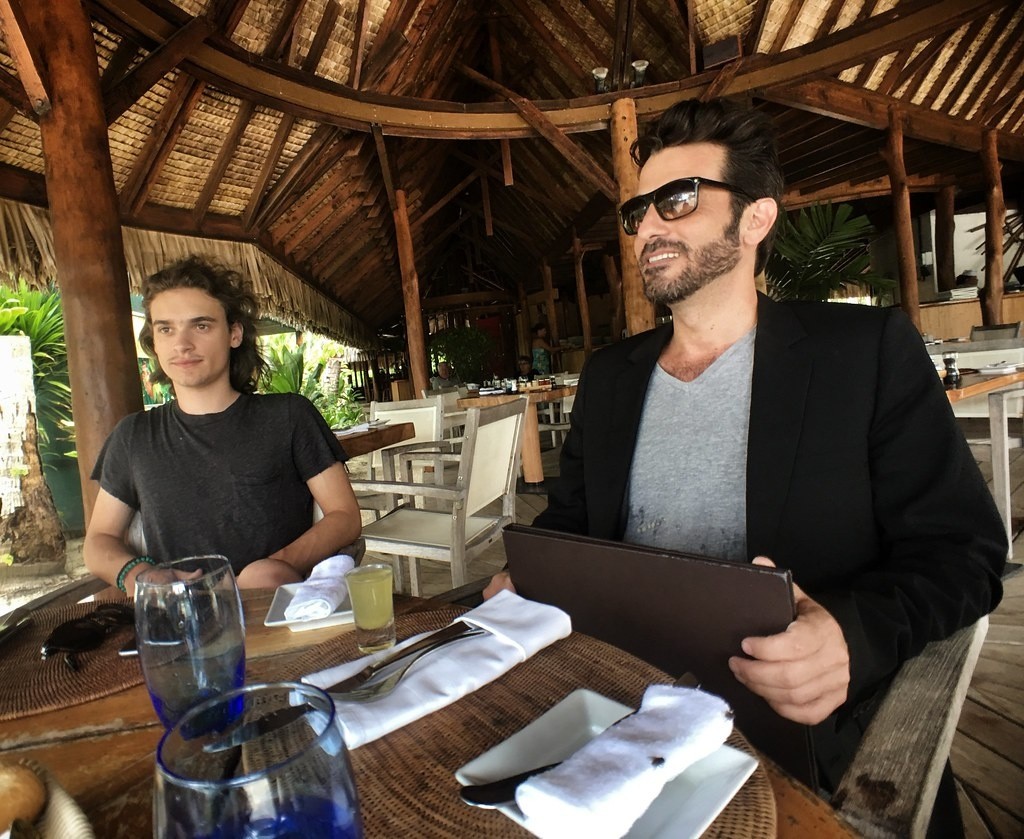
[115,557,157,593]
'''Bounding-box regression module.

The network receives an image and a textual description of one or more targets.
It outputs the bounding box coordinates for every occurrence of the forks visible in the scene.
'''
[333,627,493,703]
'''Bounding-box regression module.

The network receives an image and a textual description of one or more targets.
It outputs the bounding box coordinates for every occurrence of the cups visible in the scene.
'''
[151,681,364,839]
[559,340,566,345]
[345,564,397,654]
[134,554,247,740]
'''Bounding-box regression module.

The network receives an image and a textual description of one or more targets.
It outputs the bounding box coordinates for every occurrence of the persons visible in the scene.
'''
[482,96,1011,807]
[81,256,364,600]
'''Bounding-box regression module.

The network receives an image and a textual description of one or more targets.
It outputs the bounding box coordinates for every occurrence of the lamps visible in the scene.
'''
[629,60,649,89]
[592,66,609,94]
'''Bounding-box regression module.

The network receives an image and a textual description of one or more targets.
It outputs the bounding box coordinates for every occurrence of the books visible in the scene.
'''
[501,523,820,793]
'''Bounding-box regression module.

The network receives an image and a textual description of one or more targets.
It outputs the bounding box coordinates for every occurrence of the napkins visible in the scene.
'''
[283,554,355,634]
[290,589,574,757]
[515,684,734,838]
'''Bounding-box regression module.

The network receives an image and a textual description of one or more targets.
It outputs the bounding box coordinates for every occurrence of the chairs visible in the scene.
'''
[0,320,1024,839]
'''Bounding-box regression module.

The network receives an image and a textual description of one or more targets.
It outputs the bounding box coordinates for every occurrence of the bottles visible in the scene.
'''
[501,376,557,395]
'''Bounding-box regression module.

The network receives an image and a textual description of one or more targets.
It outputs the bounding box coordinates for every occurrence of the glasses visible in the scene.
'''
[617,175,758,236]
[40,601,134,674]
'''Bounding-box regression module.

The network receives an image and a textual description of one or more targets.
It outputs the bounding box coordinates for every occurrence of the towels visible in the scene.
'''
[515,684,734,839]
[283,554,355,622]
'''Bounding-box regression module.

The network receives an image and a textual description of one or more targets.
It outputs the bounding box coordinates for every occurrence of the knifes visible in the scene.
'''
[323,621,493,693]
[204,703,319,752]
[460,708,640,809]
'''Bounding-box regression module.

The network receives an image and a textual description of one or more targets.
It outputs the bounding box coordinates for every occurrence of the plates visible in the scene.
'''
[985,362,1024,368]
[264,582,355,633]
[455,688,759,839]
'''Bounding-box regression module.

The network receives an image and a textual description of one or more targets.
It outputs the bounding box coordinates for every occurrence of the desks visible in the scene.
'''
[336,422,415,459]
[458,387,577,493]
[938,368,1024,403]
[0,588,865,838]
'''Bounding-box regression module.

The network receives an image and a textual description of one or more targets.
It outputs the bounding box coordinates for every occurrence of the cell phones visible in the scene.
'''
[118,593,218,657]
